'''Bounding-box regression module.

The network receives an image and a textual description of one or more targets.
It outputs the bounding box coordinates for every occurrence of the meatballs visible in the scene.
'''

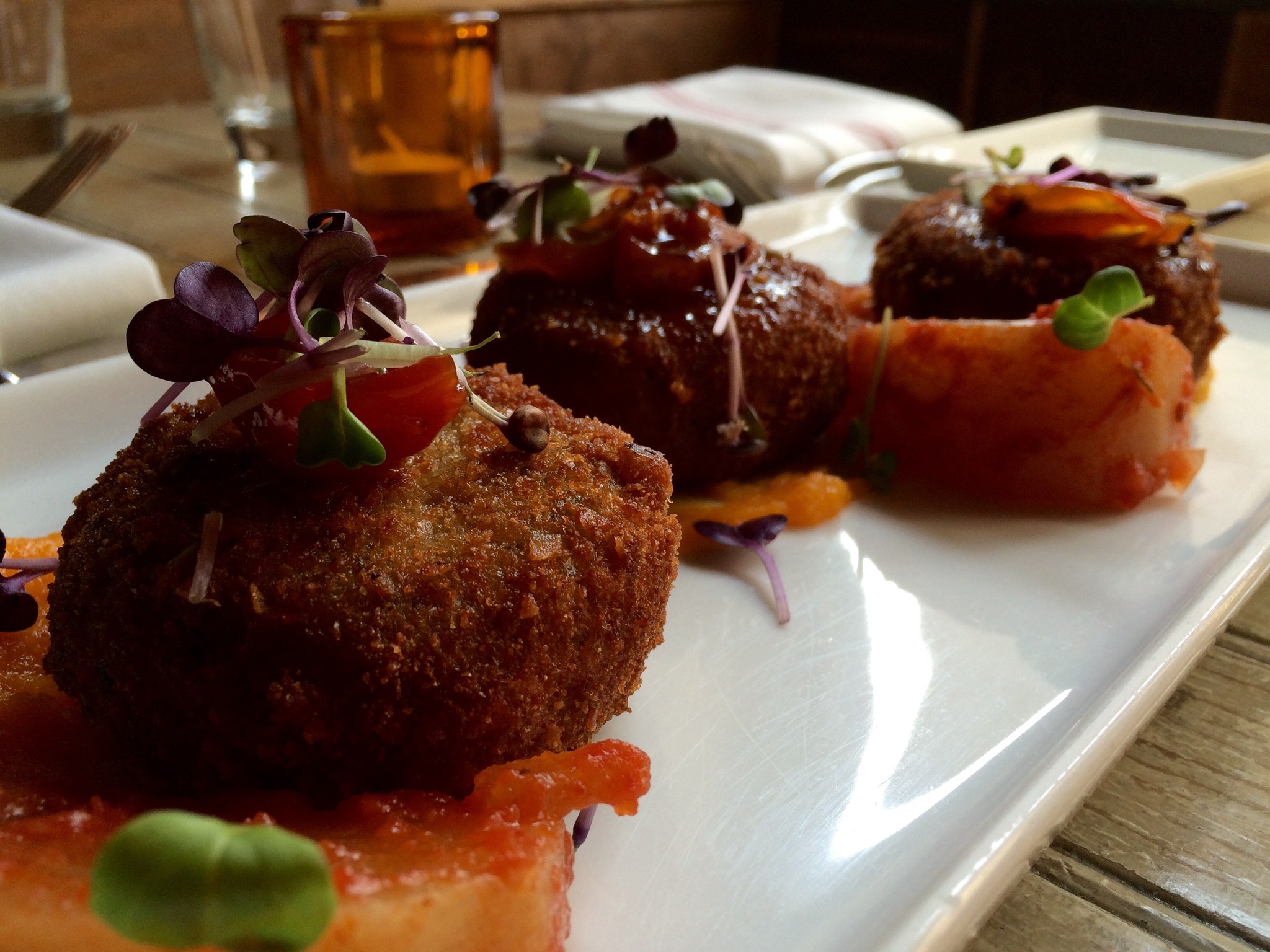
[40,360,682,801]
[468,217,853,489]
[865,187,1230,381]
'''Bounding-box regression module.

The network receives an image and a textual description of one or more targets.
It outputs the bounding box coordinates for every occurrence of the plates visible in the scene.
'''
[3,192,1270,949]
[829,109,1268,312]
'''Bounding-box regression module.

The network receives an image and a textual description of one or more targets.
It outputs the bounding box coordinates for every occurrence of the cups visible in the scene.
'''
[185,0,309,160]
[281,3,506,267]
